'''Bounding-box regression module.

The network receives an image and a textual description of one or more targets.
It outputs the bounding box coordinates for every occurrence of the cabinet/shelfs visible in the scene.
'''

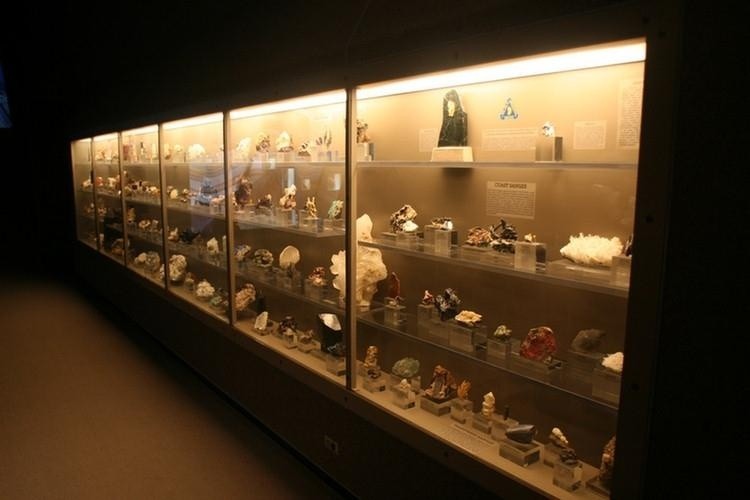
[227,89,352,390]
[70,138,98,252]
[352,38,648,500]
[159,111,233,327]
[120,123,169,289]
[92,132,128,268]
